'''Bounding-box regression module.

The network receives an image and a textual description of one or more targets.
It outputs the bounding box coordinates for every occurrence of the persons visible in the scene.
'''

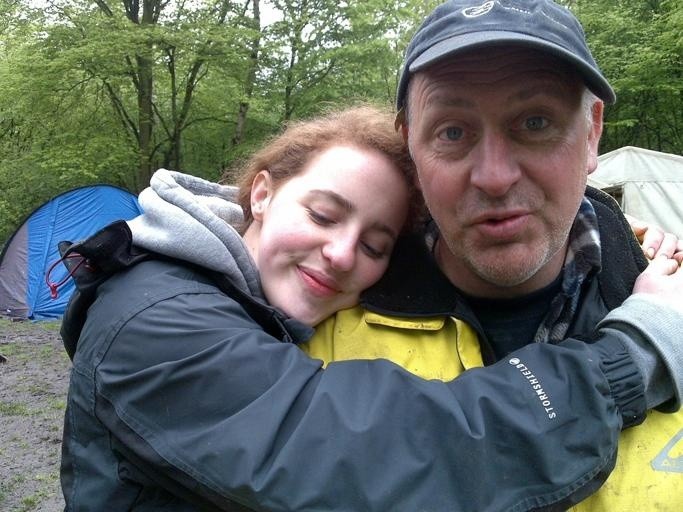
[54,103,683,510]
[289,2,683,507]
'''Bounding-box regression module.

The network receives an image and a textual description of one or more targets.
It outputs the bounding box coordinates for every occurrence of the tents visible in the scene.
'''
[0,182,150,325]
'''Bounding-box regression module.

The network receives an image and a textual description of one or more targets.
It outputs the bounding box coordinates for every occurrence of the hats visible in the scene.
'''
[393,1,617,114]
